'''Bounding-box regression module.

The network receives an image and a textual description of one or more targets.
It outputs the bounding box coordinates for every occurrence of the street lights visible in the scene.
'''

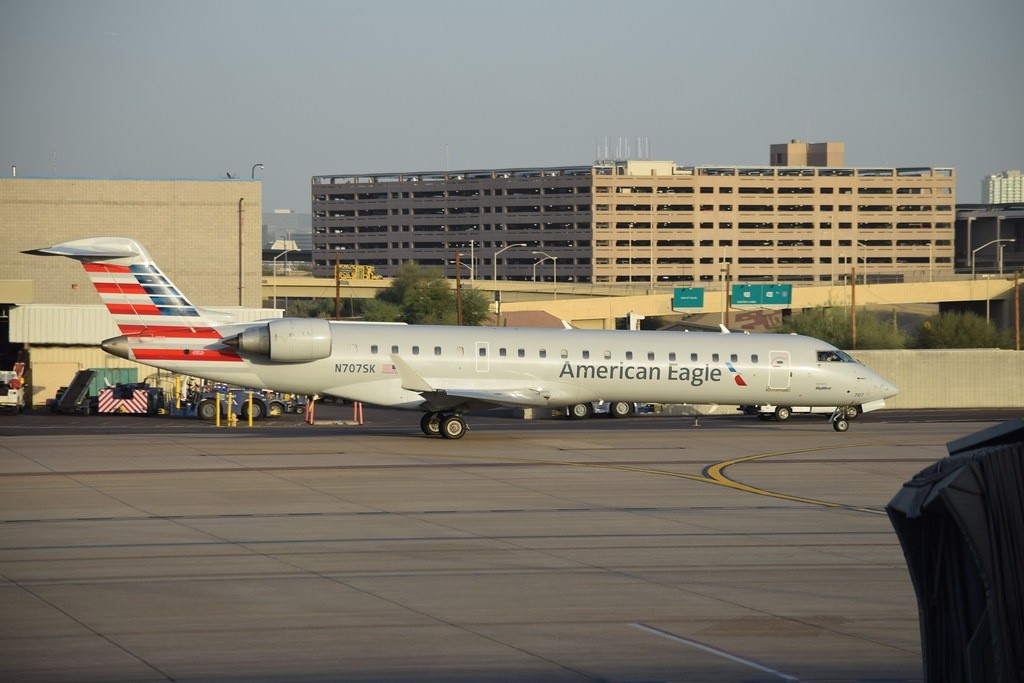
[927,242,934,283]
[531,250,557,300]
[272,248,302,308]
[279,234,288,277]
[967,215,977,267]
[628,222,634,282]
[841,234,868,284]
[720,240,729,333]
[492,242,527,289]
[971,238,1016,280]
[649,189,662,291]
[238,197,245,306]
[251,163,264,181]
[449,260,472,271]
[996,215,1006,262]
[459,252,478,281]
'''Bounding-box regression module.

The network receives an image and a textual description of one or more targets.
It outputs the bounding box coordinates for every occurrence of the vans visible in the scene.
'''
[0,370,26,415]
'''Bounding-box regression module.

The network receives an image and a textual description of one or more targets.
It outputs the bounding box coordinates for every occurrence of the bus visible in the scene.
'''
[334,264,383,280]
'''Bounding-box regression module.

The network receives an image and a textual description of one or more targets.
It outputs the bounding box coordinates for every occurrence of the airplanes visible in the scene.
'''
[20,236,899,440]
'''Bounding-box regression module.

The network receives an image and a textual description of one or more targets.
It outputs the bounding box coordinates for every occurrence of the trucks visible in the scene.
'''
[55,367,138,404]
